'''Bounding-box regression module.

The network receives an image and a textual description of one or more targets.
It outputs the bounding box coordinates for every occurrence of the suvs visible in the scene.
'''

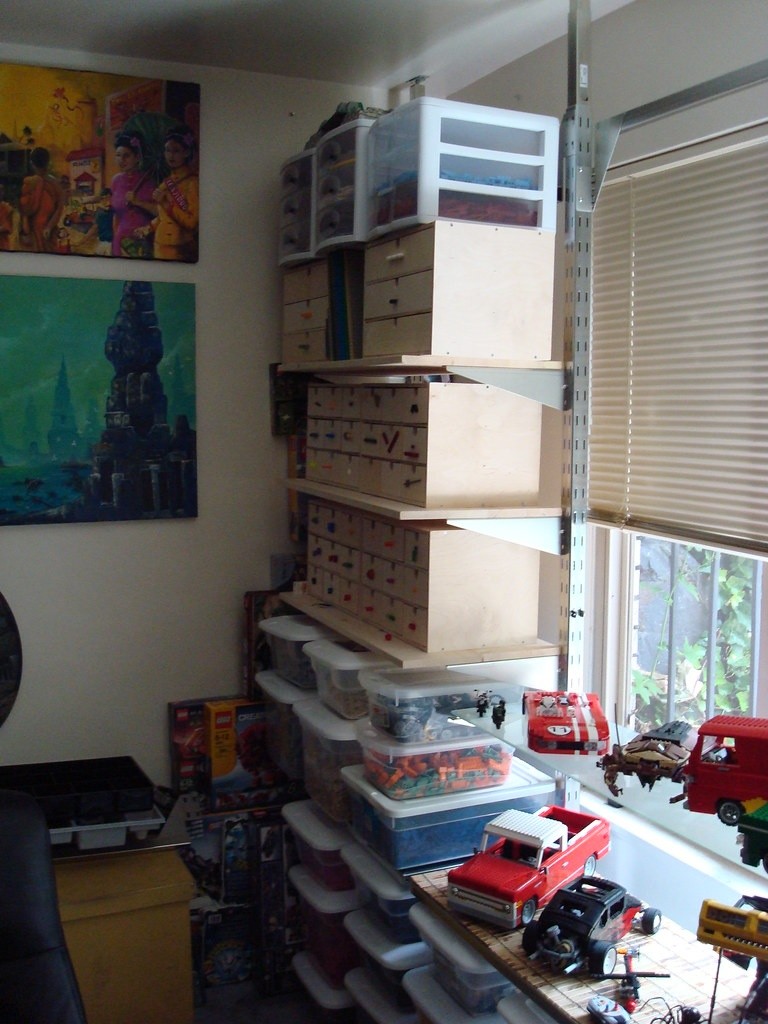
[522,875,663,981]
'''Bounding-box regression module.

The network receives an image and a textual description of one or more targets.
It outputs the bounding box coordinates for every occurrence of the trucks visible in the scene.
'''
[446,802,612,932]
[681,716,768,828]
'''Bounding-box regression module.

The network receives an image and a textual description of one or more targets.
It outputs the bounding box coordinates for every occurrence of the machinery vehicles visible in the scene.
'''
[695,895,767,1024]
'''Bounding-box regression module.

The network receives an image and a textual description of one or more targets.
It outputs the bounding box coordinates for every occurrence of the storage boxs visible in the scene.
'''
[167,98,566,1024]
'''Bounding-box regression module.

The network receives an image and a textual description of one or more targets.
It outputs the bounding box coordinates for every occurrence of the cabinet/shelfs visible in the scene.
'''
[274,355,566,669]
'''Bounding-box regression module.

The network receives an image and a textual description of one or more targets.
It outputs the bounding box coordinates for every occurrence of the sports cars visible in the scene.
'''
[521,689,612,755]
